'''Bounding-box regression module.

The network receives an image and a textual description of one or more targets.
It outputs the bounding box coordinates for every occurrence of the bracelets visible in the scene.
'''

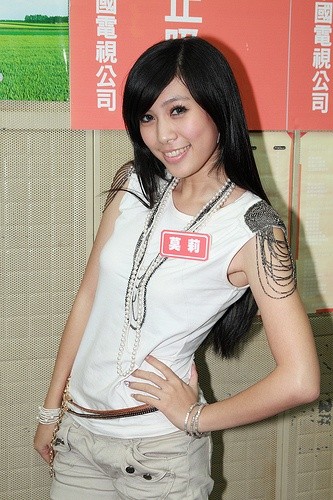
[36,402,62,425]
[184,402,211,440]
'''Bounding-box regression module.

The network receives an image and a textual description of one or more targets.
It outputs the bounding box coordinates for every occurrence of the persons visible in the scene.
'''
[34,37,320,500]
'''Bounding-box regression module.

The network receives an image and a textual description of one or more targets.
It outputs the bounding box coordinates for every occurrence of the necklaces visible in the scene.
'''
[116,175,236,378]
